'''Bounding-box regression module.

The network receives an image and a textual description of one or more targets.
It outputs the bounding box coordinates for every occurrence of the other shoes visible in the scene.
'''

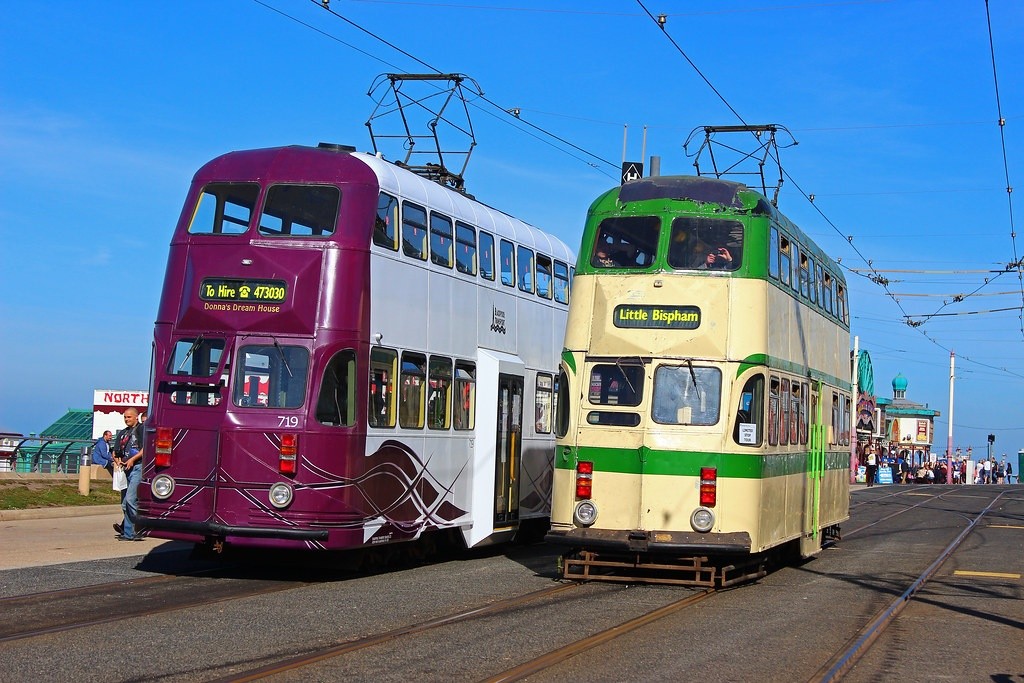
[113,523,125,536]
[133,534,144,540]
[118,534,134,541]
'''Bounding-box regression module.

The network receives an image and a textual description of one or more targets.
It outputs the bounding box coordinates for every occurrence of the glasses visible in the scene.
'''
[870,450,875,451]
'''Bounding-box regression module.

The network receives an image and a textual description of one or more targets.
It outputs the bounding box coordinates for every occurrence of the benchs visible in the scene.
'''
[91,464,112,479]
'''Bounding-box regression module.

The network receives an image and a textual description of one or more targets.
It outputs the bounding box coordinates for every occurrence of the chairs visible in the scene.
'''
[411,248,560,301]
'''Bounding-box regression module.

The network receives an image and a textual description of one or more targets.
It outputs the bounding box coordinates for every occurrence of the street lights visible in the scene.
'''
[987,434,995,484]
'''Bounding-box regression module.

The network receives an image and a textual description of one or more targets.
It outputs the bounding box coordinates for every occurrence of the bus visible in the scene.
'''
[544,124,859,591]
[136,73,578,555]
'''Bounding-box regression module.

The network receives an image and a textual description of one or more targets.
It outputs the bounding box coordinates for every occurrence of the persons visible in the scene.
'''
[113,410,148,533]
[694,234,739,270]
[974,457,1012,484]
[593,237,622,267]
[865,449,879,487]
[93,431,114,468]
[912,461,947,484]
[901,460,908,485]
[112,407,143,542]
[953,459,967,484]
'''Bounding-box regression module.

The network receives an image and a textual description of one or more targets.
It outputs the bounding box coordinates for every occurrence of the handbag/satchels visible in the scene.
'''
[112,462,128,491]
